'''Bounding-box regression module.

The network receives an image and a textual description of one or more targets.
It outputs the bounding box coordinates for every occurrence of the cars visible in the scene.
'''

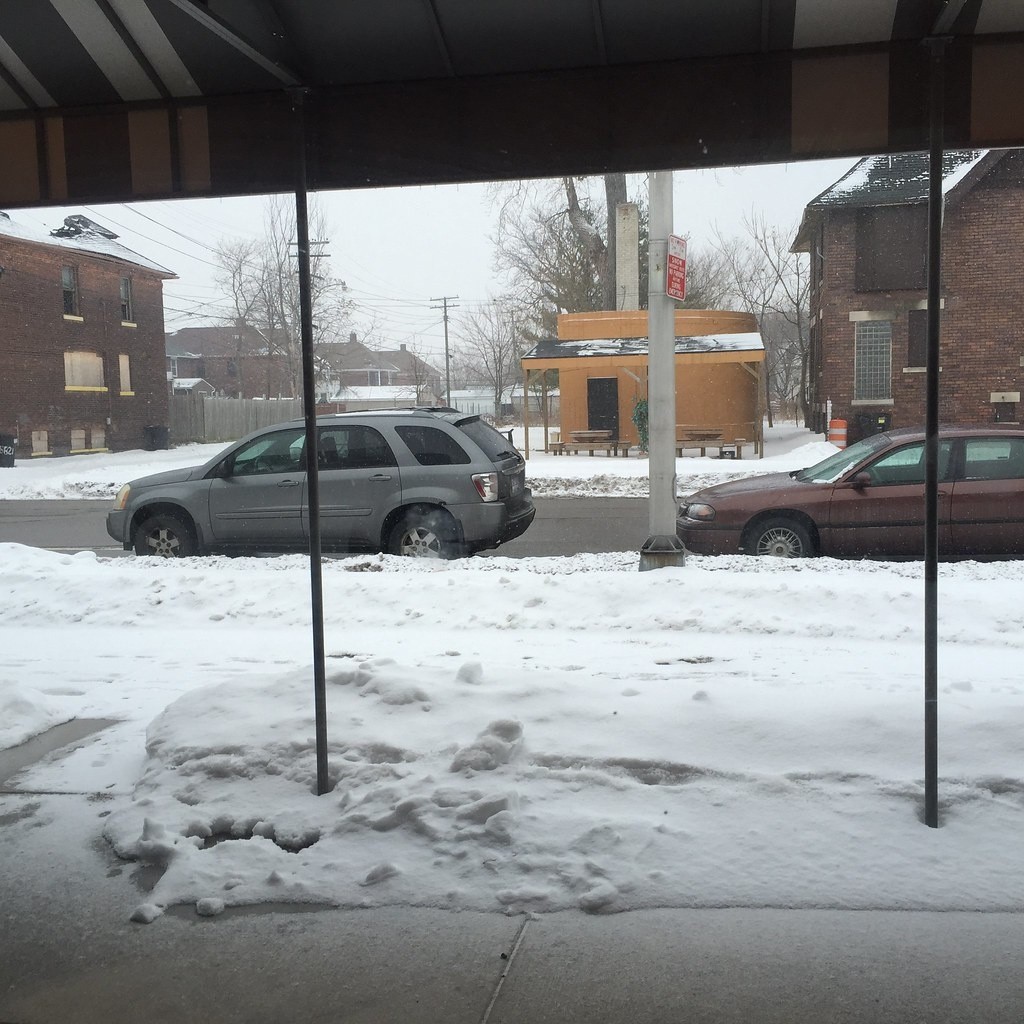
[677,421,1024,562]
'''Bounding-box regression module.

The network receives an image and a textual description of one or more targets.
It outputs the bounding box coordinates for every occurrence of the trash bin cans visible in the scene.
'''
[847,412,892,446]
[149,424,170,450]
[0,434,17,468]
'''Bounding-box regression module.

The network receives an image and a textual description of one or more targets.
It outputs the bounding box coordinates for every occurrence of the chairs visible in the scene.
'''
[321,437,338,462]
[918,445,950,479]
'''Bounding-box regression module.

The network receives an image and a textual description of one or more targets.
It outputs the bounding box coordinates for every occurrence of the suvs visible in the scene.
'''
[104,408,537,560]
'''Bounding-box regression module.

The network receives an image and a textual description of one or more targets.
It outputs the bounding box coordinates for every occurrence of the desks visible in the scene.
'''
[568,430,612,456]
[682,429,723,457]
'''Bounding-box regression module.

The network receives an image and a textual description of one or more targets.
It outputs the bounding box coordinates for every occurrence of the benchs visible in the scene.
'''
[617,441,631,457]
[549,442,564,456]
[566,440,618,457]
[735,438,746,459]
[676,438,725,461]
